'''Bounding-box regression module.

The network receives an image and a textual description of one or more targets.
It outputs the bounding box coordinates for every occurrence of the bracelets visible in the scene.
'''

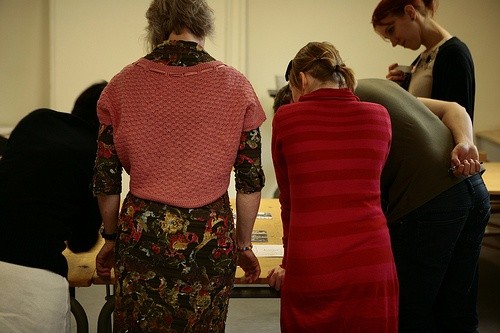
[236,244,253,252]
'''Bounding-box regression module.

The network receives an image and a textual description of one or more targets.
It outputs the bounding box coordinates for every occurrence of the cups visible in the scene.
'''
[395,66,412,92]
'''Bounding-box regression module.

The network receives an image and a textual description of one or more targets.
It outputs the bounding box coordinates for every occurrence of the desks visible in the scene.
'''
[65,198,283,333]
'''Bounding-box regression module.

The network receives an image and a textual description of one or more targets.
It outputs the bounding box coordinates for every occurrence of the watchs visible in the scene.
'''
[100,226,117,240]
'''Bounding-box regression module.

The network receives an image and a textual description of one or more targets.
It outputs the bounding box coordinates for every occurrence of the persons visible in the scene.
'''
[0,79,108,279]
[372,0,475,127]
[273,78,490,333]
[267,42,399,333]
[91,0,266,333]
